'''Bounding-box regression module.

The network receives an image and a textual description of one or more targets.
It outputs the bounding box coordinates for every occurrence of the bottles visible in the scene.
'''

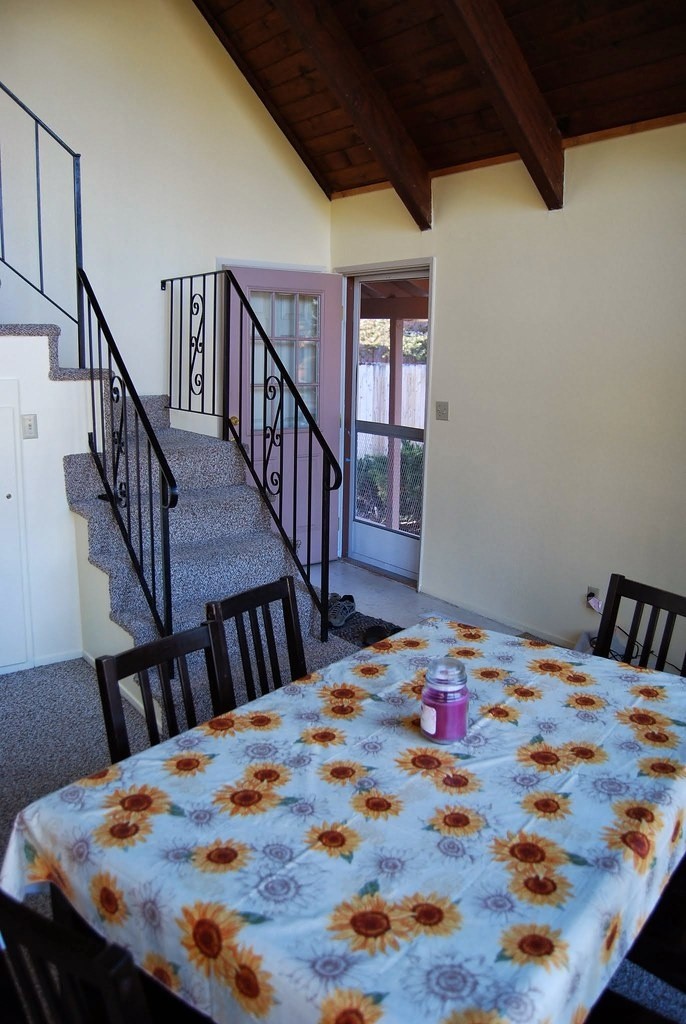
[419,659,469,745]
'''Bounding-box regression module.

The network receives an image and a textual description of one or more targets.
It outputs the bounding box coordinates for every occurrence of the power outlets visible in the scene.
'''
[585,586,601,611]
[435,401,449,422]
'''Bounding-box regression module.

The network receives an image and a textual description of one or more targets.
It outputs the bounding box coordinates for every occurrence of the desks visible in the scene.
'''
[17,612,686,1024]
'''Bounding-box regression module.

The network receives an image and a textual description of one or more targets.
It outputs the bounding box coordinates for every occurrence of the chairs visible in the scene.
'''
[0,885,136,1024]
[95,619,231,765]
[593,573,686,678]
[205,575,307,714]
